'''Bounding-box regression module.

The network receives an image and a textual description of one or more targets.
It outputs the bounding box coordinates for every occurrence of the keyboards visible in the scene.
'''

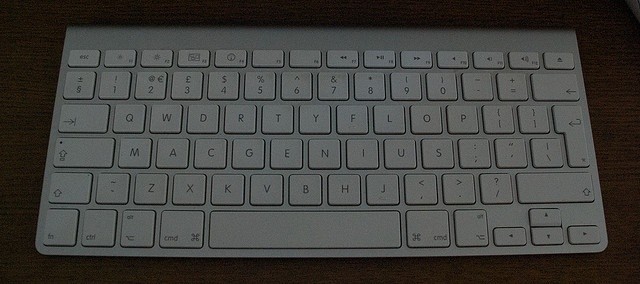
[34,24,609,256]
